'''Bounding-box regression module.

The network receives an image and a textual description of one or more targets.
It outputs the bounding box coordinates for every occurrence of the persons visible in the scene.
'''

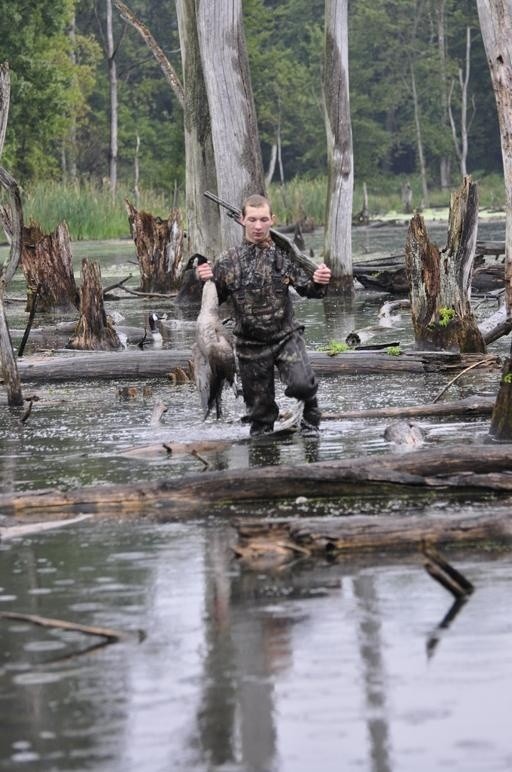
[194,194,332,436]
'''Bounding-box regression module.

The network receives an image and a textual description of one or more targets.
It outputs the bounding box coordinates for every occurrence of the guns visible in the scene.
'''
[202,190,319,279]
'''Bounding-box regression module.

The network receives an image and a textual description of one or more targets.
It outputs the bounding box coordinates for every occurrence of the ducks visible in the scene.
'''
[183,253,240,422]
[112,313,165,345]
